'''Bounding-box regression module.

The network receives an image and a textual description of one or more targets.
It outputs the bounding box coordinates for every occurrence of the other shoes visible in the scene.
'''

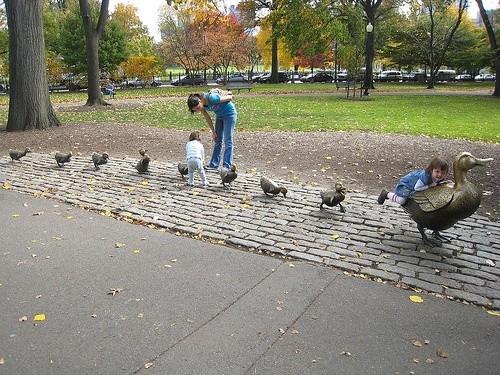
[187,182,194,186]
[203,164,229,173]
[203,181,209,186]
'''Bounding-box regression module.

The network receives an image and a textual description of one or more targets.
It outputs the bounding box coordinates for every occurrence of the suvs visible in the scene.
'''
[170,73,205,87]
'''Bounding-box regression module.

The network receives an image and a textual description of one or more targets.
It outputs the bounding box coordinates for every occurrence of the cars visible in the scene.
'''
[215,65,497,86]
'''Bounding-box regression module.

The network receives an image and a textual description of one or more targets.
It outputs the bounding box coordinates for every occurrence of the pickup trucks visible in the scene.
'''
[121,76,162,87]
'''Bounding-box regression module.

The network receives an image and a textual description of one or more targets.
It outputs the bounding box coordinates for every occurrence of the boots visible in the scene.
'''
[377,189,389,205]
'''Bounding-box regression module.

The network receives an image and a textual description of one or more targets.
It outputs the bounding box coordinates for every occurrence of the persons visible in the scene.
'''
[185,131,210,187]
[188,91,237,173]
[377,157,449,205]
[105,81,116,99]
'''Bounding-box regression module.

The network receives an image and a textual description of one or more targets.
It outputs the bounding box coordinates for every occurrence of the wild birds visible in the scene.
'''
[320,183,346,213]
[400,151,494,247]
[131,149,152,174]
[218,162,237,190]
[8,147,33,164]
[92,152,110,169]
[54,152,72,167]
[178,163,190,178]
[260,175,288,198]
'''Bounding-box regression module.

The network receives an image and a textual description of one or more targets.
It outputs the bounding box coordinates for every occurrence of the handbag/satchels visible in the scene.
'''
[207,88,232,106]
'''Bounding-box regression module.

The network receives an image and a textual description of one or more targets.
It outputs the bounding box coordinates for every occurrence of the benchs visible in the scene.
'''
[100,84,114,98]
[227,82,252,94]
[336,81,357,91]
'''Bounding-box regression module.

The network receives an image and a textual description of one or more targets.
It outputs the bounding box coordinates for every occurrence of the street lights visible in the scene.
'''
[363,22,373,96]
